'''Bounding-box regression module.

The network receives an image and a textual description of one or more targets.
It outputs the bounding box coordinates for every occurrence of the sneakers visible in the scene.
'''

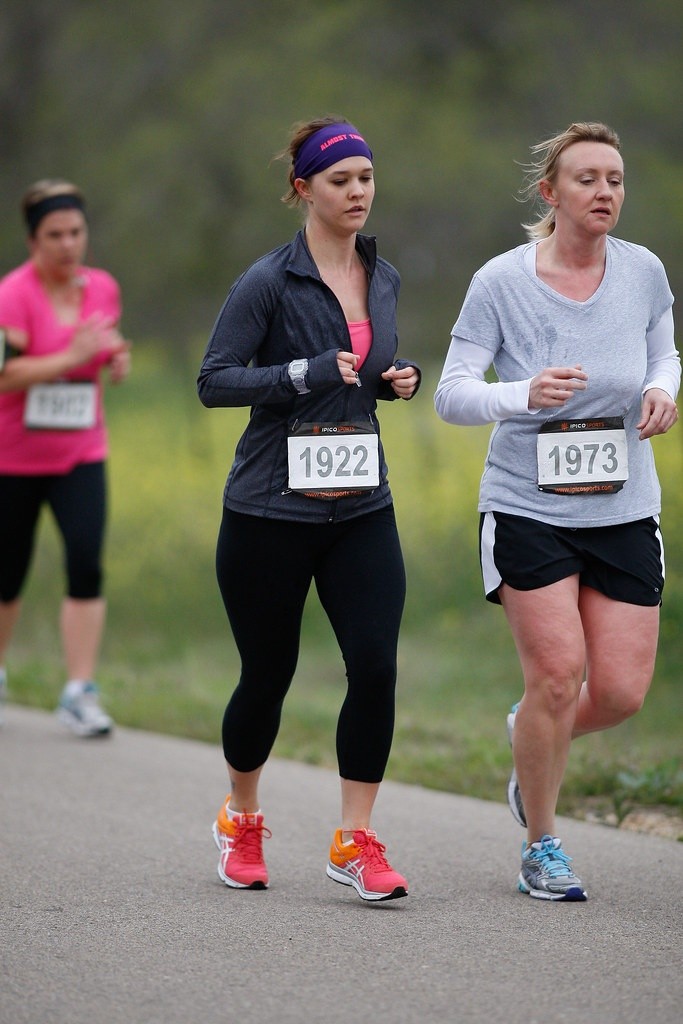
[212,794,270,890]
[59,681,112,737]
[507,702,527,827]
[327,828,409,901]
[518,834,588,901]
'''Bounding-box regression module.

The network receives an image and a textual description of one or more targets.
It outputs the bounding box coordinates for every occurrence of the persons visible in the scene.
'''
[434,119,682,901]
[0,178,134,745]
[194,115,423,902]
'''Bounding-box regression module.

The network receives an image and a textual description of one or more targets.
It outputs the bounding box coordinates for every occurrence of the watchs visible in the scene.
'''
[288,358,311,394]
